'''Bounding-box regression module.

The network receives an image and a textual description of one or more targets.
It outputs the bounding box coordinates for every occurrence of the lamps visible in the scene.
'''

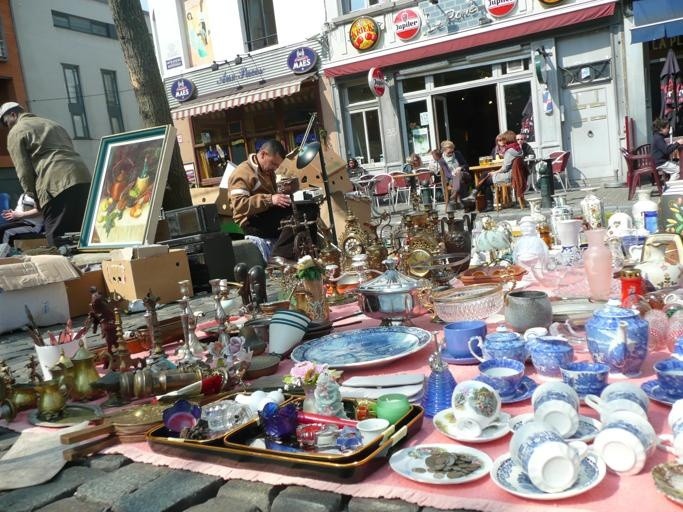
[209,60,231,74]
[426,1,460,35]
[295,109,339,248]
[233,52,253,68]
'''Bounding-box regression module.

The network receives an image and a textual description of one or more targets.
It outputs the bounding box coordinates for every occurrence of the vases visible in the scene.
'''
[578,186,605,229]
[299,380,318,417]
[581,228,614,302]
[630,189,660,236]
[548,192,573,246]
[658,178,683,246]
[523,197,547,228]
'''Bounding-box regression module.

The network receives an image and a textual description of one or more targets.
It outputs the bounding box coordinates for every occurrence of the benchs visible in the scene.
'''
[182,238,295,294]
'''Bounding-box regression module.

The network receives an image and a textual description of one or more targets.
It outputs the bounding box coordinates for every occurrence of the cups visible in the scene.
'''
[655,401,683,461]
[478,156,493,166]
[653,359,683,395]
[592,412,661,473]
[136,175,148,191]
[266,306,310,357]
[442,320,488,356]
[508,423,590,493]
[355,417,390,445]
[530,383,581,437]
[477,359,526,399]
[560,361,608,407]
[586,383,652,421]
[207,404,251,429]
[452,382,510,436]
[0,192,9,216]
[34,332,86,383]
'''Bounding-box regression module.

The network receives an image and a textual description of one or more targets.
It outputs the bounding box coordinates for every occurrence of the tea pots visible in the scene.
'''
[467,327,533,365]
[8,339,111,420]
[106,157,135,201]
[585,306,651,381]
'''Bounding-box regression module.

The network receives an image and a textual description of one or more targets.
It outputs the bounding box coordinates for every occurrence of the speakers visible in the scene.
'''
[156,232,237,294]
[165,203,220,239]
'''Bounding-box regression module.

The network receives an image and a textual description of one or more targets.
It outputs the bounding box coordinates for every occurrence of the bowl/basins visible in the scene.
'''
[244,317,269,343]
[161,399,202,435]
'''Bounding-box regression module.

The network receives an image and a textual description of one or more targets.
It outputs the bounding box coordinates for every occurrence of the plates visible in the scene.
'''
[241,354,280,380]
[438,345,481,364]
[493,452,607,497]
[650,463,683,504]
[431,408,513,445]
[642,380,683,406]
[473,377,539,403]
[390,446,491,486]
[289,326,430,369]
[508,412,600,443]
[389,267,522,322]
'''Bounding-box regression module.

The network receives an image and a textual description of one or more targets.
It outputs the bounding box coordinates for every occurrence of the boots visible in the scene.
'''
[484,200,494,212]
[461,189,479,202]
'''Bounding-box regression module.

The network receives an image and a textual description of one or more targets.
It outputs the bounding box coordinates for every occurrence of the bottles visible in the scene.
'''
[531,335,576,380]
[520,187,659,304]
[421,363,459,417]
[495,153,500,163]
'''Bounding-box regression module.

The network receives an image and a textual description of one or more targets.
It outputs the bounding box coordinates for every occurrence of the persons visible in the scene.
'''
[227,139,319,260]
[649,118,683,181]
[346,158,369,191]
[0,102,91,249]
[2,193,44,257]
[403,130,536,214]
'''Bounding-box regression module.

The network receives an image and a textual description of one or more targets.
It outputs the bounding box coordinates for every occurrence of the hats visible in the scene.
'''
[0,101,23,119]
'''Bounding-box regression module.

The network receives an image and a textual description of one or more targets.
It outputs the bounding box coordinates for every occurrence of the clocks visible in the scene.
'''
[337,204,373,274]
[395,229,449,293]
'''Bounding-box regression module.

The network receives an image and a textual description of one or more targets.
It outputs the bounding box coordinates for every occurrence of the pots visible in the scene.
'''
[504,291,552,334]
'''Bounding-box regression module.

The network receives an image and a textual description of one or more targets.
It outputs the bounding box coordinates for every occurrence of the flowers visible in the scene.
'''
[276,359,344,390]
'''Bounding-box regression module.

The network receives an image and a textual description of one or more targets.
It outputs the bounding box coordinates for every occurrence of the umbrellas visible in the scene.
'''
[659,48,683,132]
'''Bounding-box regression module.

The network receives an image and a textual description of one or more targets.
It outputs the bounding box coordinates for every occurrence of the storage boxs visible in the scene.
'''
[316,166,354,199]
[0,234,197,340]
[273,140,348,195]
[313,186,372,257]
[186,186,234,218]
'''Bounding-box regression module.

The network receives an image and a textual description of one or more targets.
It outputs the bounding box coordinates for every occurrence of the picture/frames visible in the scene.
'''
[75,123,179,254]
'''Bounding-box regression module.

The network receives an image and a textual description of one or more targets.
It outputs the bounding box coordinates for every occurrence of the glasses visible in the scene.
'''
[445,150,455,154]
[517,139,524,141]
